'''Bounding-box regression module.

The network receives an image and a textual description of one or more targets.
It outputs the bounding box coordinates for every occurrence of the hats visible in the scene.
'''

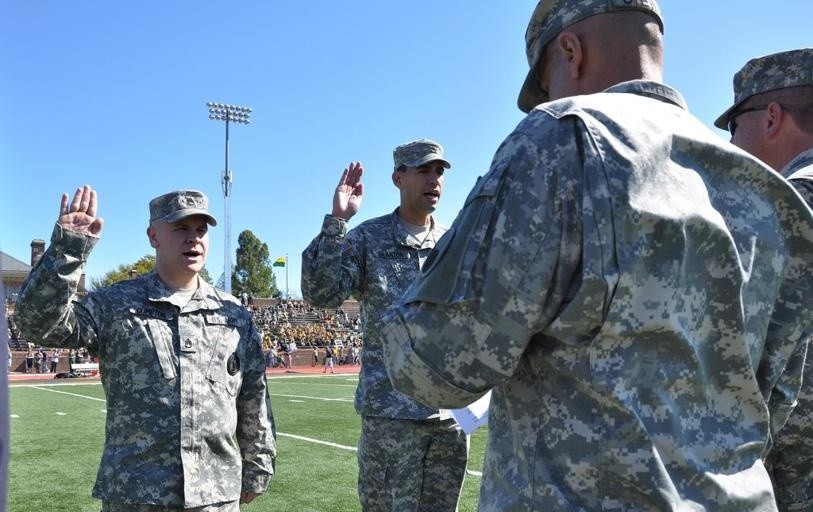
[392,138,452,170]
[148,189,218,228]
[712,46,813,130]
[517,0,665,115]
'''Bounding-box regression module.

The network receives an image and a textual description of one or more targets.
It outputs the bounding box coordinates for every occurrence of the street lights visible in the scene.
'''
[205,101,253,298]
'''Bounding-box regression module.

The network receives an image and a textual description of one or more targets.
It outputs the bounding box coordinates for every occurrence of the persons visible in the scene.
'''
[236,292,362,374]
[296,136,479,511]
[5,295,20,341]
[11,183,279,512]
[69,347,102,373]
[374,0,813,512]
[26,347,59,374]
[710,47,812,511]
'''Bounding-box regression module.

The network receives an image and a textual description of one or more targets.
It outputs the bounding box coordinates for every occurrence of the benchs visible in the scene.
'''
[4,284,30,350]
[241,298,360,346]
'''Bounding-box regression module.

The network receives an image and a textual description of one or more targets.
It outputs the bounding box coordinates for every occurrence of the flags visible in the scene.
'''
[272,255,285,268]
